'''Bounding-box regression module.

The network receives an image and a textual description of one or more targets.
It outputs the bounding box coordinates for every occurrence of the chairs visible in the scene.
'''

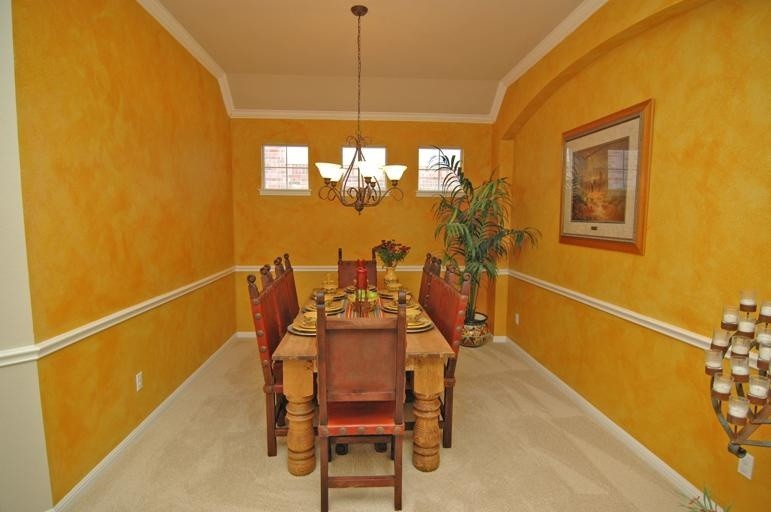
[389,250,456,462]
[336,250,378,288]
[310,289,411,511]
[247,249,318,459]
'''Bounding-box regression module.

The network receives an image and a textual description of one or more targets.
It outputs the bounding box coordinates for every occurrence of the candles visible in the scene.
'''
[702,293,771,432]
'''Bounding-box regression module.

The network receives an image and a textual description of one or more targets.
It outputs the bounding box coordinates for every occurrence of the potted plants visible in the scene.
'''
[427,145,540,348]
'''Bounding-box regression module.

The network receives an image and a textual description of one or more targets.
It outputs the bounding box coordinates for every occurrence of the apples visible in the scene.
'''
[351,290,376,299]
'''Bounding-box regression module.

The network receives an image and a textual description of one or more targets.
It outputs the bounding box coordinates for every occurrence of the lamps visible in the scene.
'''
[312,5,406,212]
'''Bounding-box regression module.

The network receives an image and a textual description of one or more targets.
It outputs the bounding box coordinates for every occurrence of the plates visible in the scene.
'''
[293,290,346,332]
[379,289,431,328]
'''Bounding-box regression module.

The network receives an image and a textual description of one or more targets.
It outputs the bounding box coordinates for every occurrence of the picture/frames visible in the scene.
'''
[559,97,656,255]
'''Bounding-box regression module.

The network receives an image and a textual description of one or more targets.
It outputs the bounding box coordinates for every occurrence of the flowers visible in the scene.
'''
[374,239,411,267]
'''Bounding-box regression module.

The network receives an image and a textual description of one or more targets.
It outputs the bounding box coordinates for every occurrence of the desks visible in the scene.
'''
[270,285,458,478]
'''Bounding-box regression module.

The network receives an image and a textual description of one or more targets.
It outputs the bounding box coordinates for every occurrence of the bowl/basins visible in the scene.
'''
[323,286,338,295]
[386,284,402,292]
